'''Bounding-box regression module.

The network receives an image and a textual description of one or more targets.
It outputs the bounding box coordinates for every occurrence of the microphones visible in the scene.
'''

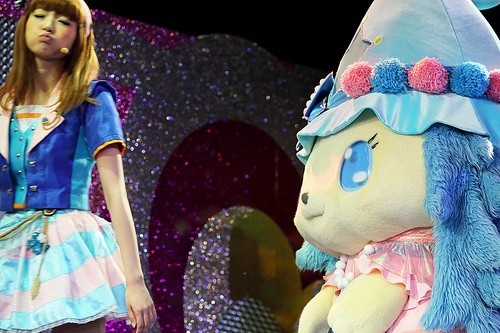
[60,47,70,54]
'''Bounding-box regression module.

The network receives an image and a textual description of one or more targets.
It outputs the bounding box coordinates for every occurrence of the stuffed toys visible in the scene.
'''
[291,0,500,333]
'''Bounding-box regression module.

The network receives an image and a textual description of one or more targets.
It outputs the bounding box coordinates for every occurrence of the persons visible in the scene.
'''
[0,0,157,333]
